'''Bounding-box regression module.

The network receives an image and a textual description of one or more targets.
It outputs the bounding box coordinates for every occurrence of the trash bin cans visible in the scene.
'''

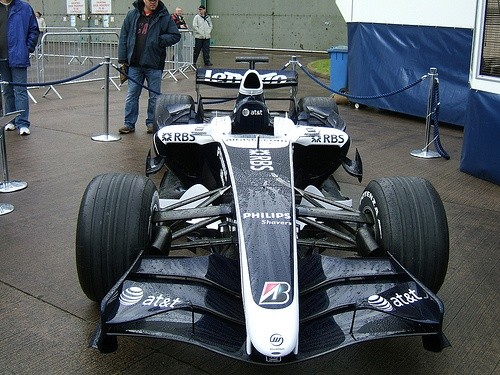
[327,44,349,94]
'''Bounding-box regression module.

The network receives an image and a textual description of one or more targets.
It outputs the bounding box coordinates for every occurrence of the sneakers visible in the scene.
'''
[5,123,31,136]
[119,126,136,133]
[147,124,154,133]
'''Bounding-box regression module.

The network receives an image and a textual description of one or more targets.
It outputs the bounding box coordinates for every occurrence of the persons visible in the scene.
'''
[36,11,47,51]
[171,7,188,71]
[192,5,213,71]
[118,0,182,133]
[0,0,40,135]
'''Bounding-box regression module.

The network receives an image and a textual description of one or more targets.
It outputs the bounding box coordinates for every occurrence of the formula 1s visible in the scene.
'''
[76,54,450,366]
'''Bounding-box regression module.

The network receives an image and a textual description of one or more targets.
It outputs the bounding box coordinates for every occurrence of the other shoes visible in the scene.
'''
[204,62,214,67]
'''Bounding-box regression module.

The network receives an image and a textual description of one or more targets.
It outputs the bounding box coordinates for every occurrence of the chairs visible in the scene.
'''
[231,56,273,136]
